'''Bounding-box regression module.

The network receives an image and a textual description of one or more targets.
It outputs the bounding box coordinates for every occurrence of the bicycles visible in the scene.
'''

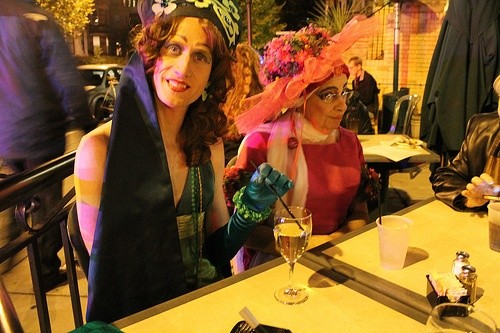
[86,75,120,124]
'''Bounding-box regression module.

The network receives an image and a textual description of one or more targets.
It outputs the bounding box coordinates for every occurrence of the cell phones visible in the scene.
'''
[229,320,291,333]
[482,194,500,201]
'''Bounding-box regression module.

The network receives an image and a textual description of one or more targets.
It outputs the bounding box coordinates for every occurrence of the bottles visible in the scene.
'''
[449,251,476,303]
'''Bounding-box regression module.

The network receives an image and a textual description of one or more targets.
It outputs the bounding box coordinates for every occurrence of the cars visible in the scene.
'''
[76,63,125,122]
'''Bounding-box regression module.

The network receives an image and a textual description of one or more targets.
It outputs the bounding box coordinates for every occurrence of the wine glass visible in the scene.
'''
[274,205,312,306]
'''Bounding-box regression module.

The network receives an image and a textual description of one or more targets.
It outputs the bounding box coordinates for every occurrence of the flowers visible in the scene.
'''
[260,21,334,84]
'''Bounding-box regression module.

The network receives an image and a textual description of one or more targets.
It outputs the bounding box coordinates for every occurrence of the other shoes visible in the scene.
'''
[40,269,69,292]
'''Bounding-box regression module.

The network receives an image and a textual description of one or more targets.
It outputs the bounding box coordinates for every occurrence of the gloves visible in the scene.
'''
[240,163,293,214]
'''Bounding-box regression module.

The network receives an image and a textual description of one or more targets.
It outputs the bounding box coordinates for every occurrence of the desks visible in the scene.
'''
[300,196,500,333]
[356,134,440,217]
[110,256,467,333]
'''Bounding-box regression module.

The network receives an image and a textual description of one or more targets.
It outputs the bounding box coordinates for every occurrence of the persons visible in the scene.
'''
[224,15,383,274]
[432,74,500,211]
[421,0,500,179]
[0,0,88,292]
[339,56,379,134]
[74,0,294,323]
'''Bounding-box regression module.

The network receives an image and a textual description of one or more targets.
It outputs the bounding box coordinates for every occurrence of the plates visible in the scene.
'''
[394,139,423,149]
[67,319,123,333]
[230,320,292,333]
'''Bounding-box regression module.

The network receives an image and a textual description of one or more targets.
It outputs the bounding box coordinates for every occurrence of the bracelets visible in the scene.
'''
[232,185,271,221]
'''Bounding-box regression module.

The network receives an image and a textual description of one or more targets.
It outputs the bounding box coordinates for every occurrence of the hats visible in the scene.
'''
[139,0,245,55]
[233,5,376,134]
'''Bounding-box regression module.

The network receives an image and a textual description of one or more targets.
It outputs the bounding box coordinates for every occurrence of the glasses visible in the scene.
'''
[309,90,354,104]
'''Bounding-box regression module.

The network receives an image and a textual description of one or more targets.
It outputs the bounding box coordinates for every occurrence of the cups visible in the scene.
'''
[345,118,359,135]
[487,201,500,252]
[425,302,497,333]
[376,214,412,271]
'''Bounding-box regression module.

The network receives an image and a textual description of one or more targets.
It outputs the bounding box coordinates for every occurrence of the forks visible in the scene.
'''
[236,322,253,333]
